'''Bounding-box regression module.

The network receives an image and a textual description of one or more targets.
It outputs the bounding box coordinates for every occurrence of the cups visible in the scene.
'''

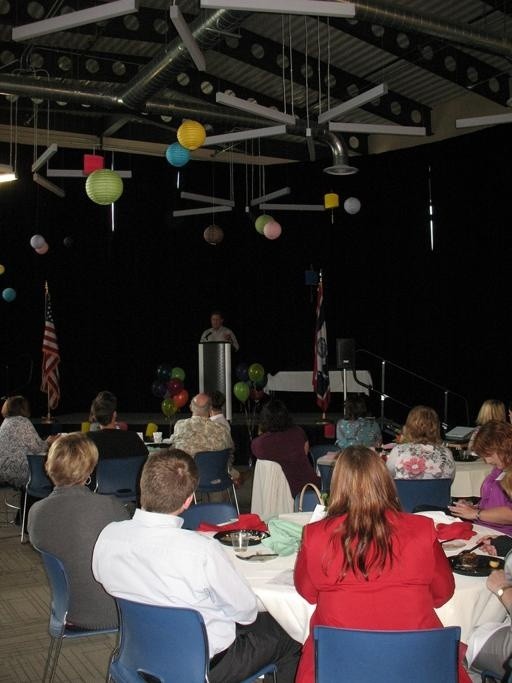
[81,422,90,432]
[325,424,335,439]
[231,532,250,552]
[152,431,163,443]
[145,423,159,439]
[375,441,383,458]
[137,432,143,440]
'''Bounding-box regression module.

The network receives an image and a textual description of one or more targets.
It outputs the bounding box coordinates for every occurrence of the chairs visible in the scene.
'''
[295,492,320,512]
[32,543,119,682]
[190,447,241,515]
[307,621,463,683]
[92,459,144,503]
[20,454,56,544]
[105,596,211,683]
[391,478,454,512]
[178,505,237,530]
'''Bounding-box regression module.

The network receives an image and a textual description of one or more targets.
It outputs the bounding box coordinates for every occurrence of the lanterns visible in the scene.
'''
[344,197,361,215]
[263,220,282,239]
[255,215,274,235]
[166,142,192,167]
[85,169,123,206]
[204,224,224,245]
[0,234,49,303]
[177,119,206,151]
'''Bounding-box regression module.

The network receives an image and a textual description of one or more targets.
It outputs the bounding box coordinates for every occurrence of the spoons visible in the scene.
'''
[457,536,492,557]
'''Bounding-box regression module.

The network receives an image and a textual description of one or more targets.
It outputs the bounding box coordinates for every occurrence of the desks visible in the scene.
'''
[187,508,512,650]
[58,433,177,459]
[316,445,493,493]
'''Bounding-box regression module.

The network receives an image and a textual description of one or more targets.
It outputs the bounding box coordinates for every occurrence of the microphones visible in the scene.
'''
[204,332,212,338]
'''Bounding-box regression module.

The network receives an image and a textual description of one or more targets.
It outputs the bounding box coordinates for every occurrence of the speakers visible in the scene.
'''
[335,338,355,369]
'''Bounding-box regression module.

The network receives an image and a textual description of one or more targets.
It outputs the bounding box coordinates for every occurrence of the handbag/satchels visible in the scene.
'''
[278,511,330,525]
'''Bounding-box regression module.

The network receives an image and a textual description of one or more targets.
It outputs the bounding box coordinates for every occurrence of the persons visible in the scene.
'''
[250,399,322,502]
[92,448,302,683]
[27,432,132,630]
[471,535,512,683]
[293,445,473,683]
[88,393,234,504]
[335,394,456,489]
[200,312,240,353]
[446,398,512,535]
[1,394,61,489]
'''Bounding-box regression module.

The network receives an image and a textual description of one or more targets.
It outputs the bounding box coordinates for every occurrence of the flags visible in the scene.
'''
[312,282,330,411]
[40,293,62,410]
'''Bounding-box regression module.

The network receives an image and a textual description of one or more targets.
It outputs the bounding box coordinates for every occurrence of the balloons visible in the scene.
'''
[249,364,265,383]
[151,362,188,421]
[234,382,250,402]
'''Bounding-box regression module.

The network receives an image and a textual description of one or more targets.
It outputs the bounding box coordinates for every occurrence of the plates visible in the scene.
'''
[213,528,270,546]
[452,450,480,462]
[442,539,467,550]
[447,553,504,577]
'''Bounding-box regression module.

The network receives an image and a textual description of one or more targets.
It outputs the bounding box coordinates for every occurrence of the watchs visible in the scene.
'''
[498,586,512,599]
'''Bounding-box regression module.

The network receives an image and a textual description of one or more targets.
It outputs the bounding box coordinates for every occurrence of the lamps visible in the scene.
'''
[0,0,512,253]
